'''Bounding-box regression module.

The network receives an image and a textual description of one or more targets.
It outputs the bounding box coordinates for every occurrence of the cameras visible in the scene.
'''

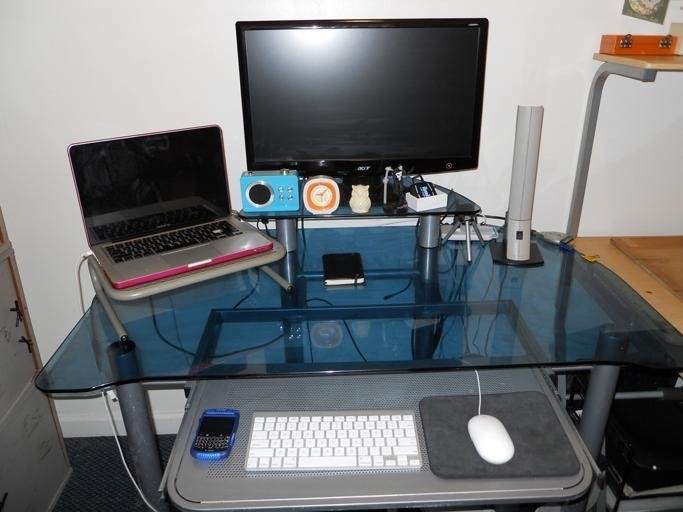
[239,168,300,213]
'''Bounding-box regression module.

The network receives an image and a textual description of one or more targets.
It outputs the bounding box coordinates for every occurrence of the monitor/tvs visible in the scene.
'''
[235,18,489,207]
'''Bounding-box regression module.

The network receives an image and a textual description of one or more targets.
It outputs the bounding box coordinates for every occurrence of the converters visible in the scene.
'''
[441,224,494,242]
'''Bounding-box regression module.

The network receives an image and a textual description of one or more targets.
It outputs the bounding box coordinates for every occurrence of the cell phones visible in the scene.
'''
[192,407,239,460]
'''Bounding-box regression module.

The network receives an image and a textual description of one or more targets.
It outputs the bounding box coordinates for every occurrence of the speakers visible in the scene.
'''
[488,105,545,267]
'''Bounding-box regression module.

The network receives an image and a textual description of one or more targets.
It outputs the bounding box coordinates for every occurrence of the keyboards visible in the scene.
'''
[244,409,423,470]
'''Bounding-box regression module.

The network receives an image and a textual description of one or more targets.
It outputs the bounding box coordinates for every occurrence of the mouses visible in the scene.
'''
[467,415,514,465]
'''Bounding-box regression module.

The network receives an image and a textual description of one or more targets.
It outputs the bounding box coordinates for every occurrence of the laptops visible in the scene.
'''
[67,125,274,290]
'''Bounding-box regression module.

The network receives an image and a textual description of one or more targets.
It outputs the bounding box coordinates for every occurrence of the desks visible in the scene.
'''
[32,224,683,512]
